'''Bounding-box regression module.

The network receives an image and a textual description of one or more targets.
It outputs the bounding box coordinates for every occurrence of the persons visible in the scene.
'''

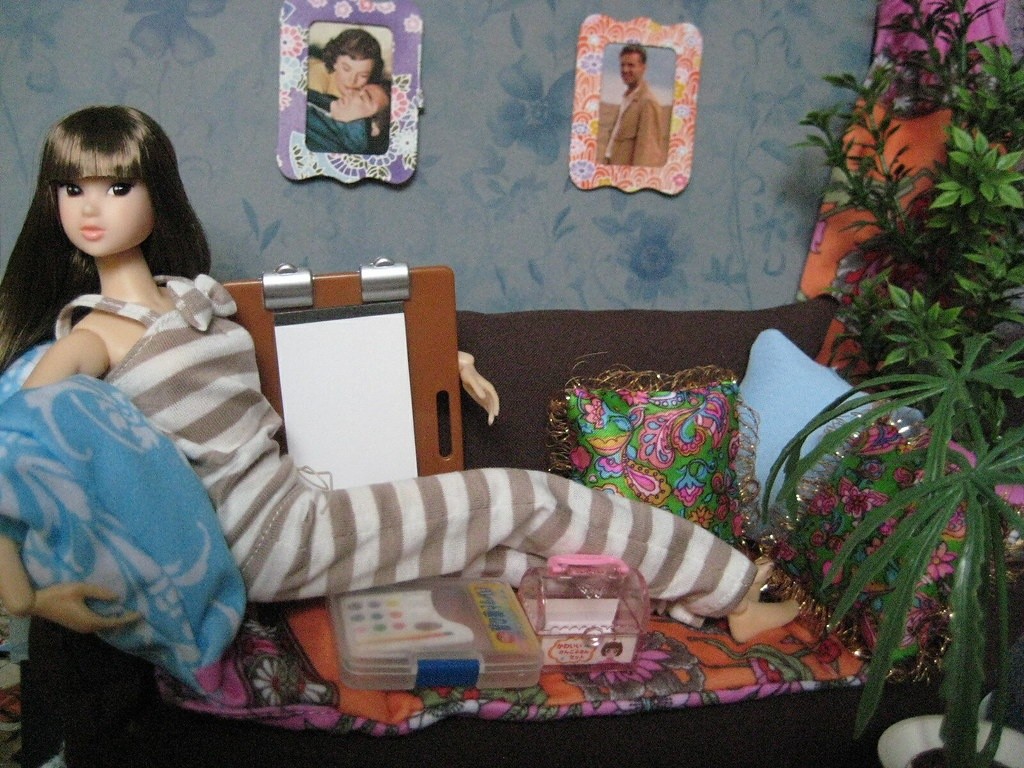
[0,106,801,643]
[604,45,663,168]
[305,28,390,155]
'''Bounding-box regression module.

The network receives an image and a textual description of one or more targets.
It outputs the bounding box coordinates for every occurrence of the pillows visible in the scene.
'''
[538,357,762,551]
[0,343,249,707]
[754,392,1023,688]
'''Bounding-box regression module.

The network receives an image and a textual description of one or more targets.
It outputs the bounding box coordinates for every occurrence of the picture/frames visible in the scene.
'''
[276,0,429,190]
[567,12,703,195]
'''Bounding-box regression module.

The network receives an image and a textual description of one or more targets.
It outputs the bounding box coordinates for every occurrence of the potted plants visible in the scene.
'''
[758,0,1024,768]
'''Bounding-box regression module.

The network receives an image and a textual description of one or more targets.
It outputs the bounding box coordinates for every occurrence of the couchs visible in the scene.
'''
[13,292,1024,768]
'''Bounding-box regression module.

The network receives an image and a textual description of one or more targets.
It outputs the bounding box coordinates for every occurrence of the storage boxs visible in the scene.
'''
[332,582,541,689]
